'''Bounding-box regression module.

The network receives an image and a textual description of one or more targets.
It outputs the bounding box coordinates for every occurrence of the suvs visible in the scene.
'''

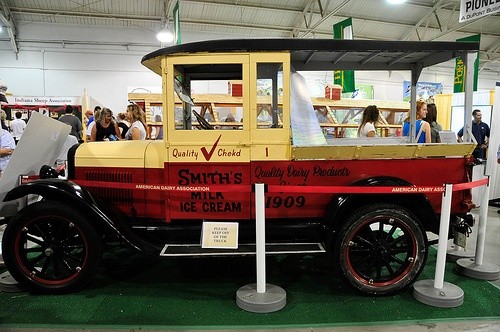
[0,34,482,297]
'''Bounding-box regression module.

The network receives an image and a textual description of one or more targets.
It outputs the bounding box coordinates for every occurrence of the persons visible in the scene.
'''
[458,109,490,160]
[0,86,8,105]
[400,102,442,143]
[0,109,26,174]
[154,115,163,139]
[318,108,335,139]
[358,105,379,137]
[57,103,148,177]
